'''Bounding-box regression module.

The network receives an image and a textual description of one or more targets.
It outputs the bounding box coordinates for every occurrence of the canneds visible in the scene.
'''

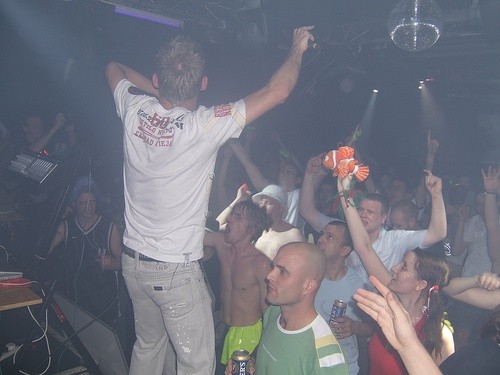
[330,300,347,329]
[231,349,250,375]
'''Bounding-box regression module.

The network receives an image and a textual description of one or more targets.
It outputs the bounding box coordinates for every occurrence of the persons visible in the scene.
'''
[197,217,221,313]
[208,122,500,276]
[223,241,348,375]
[47,186,123,331]
[298,150,449,298]
[335,172,456,375]
[352,275,442,375]
[441,270,500,310]
[215,183,306,262]
[480,304,500,347]
[0,109,124,217]
[203,199,275,375]
[103,25,316,375]
[313,221,382,375]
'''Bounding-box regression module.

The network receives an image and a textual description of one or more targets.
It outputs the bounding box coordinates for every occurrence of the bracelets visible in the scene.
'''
[338,189,351,194]
[337,193,355,207]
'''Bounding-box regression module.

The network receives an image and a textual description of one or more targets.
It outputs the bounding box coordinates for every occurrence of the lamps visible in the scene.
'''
[2,148,58,185]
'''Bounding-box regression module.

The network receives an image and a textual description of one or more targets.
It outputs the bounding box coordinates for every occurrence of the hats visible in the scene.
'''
[252,184,289,219]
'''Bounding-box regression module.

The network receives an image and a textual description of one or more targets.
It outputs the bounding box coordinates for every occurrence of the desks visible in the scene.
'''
[0,286,43,375]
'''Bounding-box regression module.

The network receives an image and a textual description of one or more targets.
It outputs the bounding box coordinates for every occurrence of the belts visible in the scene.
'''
[123,246,158,262]
